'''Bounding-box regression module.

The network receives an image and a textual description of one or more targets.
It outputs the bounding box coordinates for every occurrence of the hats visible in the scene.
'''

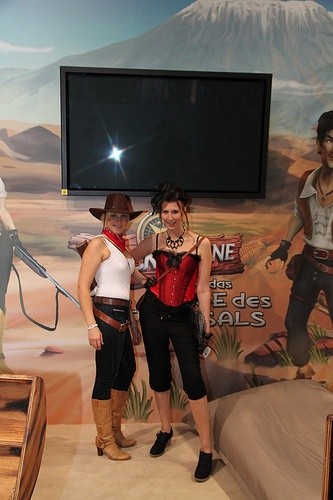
[88,193,144,221]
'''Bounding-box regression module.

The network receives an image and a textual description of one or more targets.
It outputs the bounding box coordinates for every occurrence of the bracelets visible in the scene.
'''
[87,323,98,330]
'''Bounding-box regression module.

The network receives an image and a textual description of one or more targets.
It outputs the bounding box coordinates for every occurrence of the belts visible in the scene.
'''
[94,297,131,308]
[93,305,128,333]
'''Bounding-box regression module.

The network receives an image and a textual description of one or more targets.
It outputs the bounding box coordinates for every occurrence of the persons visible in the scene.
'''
[77,194,142,460]
[129,188,213,483]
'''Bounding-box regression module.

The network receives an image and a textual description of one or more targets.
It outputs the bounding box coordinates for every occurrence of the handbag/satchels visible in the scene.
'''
[136,294,146,310]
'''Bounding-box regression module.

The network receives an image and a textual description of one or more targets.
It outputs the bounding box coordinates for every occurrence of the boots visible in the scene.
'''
[91,398,130,460]
[110,388,137,448]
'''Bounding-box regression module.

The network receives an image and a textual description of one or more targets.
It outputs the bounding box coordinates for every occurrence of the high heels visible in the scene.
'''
[194,448,213,481]
[150,426,173,457]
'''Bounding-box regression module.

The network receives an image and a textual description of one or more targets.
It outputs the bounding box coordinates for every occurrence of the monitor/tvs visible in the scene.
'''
[59,64,273,199]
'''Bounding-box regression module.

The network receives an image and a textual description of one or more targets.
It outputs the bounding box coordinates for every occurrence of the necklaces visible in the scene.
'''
[165,230,186,249]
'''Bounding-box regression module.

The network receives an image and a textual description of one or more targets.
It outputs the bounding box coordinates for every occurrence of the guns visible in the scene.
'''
[13,240,82,309]
